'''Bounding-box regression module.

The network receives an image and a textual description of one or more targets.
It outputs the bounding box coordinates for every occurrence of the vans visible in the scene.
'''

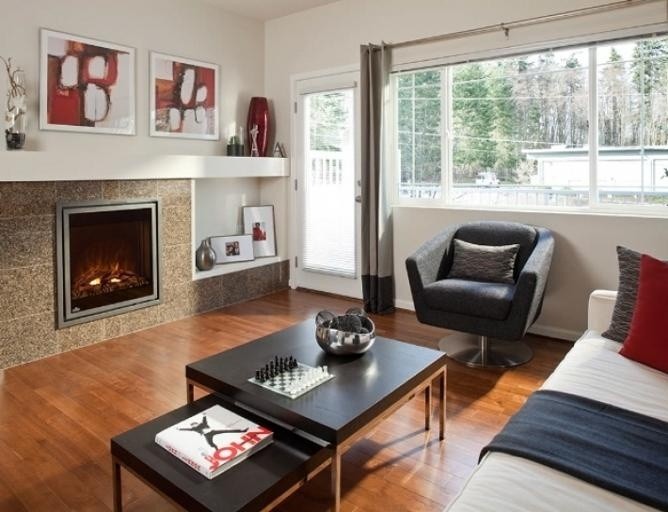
[476,172,500,188]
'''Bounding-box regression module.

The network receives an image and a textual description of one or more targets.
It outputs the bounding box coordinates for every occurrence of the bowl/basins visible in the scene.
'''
[315,313,376,355]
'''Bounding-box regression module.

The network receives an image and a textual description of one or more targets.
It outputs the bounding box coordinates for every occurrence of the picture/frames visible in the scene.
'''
[38,27,137,136]
[149,52,219,140]
[208,234,255,264]
[242,205,277,258]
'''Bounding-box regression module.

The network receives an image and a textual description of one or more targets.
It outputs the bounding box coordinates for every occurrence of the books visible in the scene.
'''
[154,404,274,481]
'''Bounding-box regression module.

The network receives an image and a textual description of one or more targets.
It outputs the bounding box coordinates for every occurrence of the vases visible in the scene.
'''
[5,132,25,150]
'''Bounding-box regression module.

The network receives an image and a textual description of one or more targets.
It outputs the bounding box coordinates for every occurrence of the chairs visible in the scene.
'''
[405,221,555,370]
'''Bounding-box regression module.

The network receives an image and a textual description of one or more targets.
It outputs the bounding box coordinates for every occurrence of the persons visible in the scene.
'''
[177,413,248,451]
[226,245,234,255]
[253,223,262,241]
[234,242,239,255]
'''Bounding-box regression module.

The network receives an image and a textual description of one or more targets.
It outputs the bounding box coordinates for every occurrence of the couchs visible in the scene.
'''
[442,245,668,512]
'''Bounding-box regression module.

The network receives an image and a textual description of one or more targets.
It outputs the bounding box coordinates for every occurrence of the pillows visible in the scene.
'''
[617,254,668,374]
[601,244,644,343]
[447,238,522,287]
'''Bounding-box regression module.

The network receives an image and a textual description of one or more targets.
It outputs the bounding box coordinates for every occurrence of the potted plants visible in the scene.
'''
[227,135,245,156]
